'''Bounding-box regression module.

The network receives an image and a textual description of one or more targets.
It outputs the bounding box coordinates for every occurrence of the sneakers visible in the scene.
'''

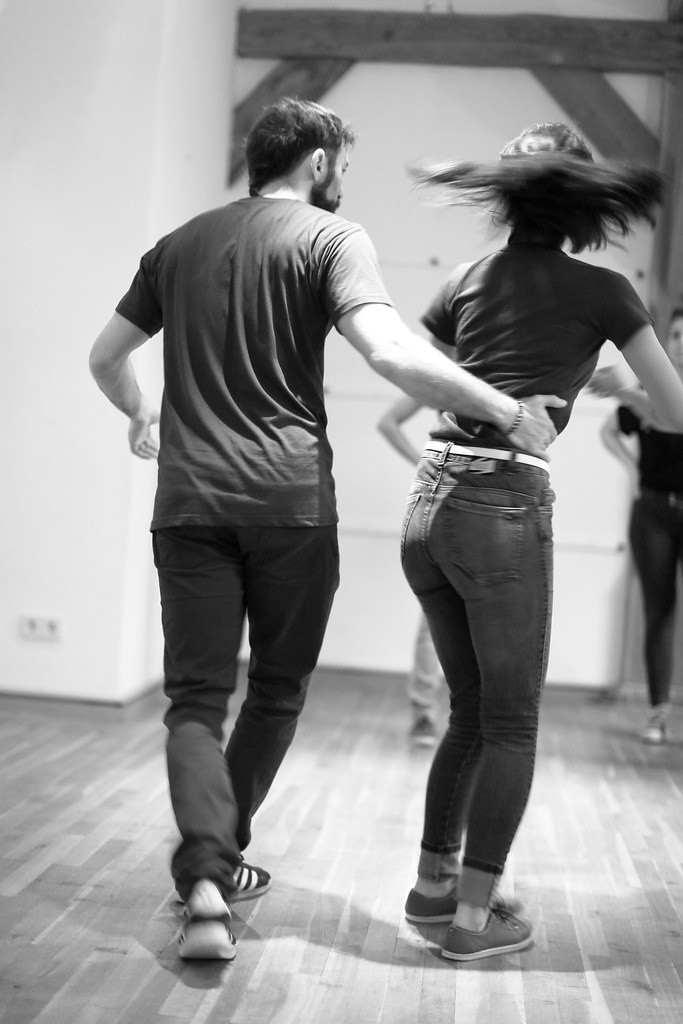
[404,887,540,962]
[168,863,274,961]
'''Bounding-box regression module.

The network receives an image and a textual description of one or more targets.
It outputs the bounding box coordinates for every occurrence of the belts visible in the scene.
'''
[424,440,551,475]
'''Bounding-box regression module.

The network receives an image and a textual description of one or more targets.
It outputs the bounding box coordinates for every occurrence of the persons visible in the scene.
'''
[90,98,566,960]
[380,124,683,961]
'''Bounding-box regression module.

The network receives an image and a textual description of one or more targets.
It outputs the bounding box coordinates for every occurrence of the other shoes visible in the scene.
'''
[412,718,438,745]
[644,716,667,744]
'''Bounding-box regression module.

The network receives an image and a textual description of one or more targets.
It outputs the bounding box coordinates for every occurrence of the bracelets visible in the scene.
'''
[504,400,524,436]
